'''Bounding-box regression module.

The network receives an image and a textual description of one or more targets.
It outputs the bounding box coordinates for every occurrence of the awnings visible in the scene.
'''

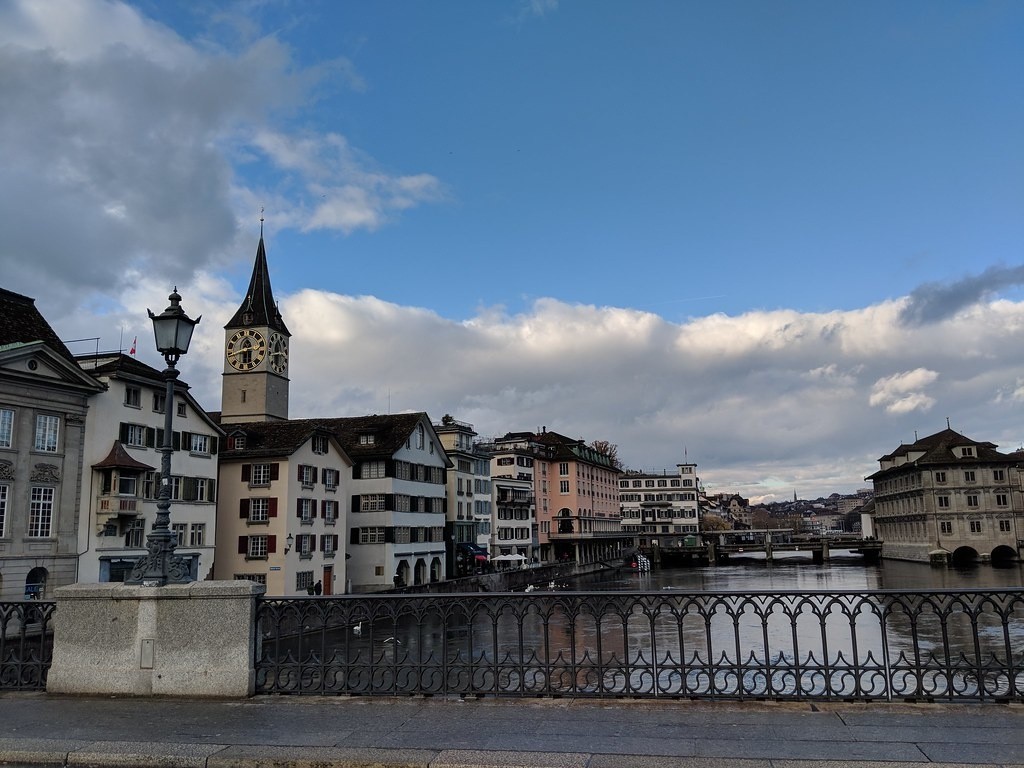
[458,543,490,556]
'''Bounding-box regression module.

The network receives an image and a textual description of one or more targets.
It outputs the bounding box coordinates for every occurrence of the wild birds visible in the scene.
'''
[353,621,362,630]
[383,637,401,644]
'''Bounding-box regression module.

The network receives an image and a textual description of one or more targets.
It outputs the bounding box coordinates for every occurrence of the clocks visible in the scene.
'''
[267,332,289,375]
[226,329,267,371]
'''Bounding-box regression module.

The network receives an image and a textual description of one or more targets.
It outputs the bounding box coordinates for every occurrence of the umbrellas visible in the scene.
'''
[475,555,486,560]
[491,554,528,561]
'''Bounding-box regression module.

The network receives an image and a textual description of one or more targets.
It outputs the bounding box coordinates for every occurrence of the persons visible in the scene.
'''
[497,560,507,572]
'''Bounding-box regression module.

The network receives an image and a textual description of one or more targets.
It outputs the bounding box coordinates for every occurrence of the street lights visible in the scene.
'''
[124,286,202,587]
